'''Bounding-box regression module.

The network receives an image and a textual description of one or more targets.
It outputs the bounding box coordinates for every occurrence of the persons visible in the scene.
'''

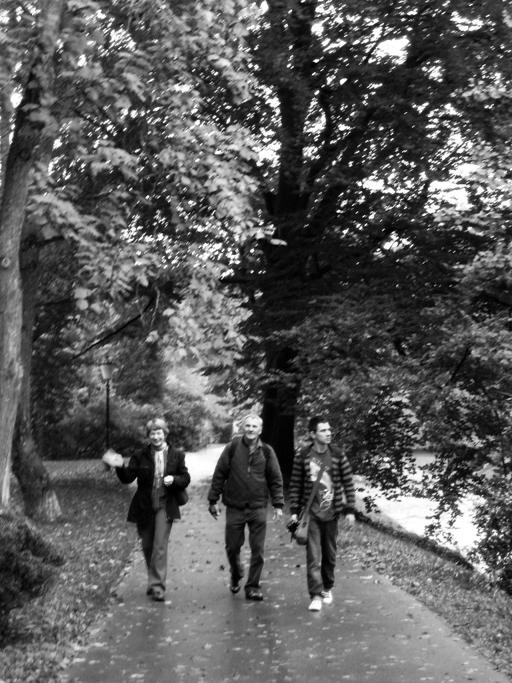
[289,415,356,612]
[111,419,189,602]
[207,413,284,601]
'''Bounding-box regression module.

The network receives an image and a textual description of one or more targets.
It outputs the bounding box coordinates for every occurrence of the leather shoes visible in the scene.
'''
[245,588,263,600]
[231,583,241,593]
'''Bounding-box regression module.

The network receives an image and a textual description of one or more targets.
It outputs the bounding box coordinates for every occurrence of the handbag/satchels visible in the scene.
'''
[292,510,312,545]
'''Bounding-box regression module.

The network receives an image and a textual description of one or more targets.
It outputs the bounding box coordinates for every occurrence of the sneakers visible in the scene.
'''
[321,589,333,604]
[146,587,165,601]
[175,485,189,507]
[307,595,323,611]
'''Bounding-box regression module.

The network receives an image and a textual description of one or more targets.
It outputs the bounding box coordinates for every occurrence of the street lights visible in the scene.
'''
[98,353,119,474]
